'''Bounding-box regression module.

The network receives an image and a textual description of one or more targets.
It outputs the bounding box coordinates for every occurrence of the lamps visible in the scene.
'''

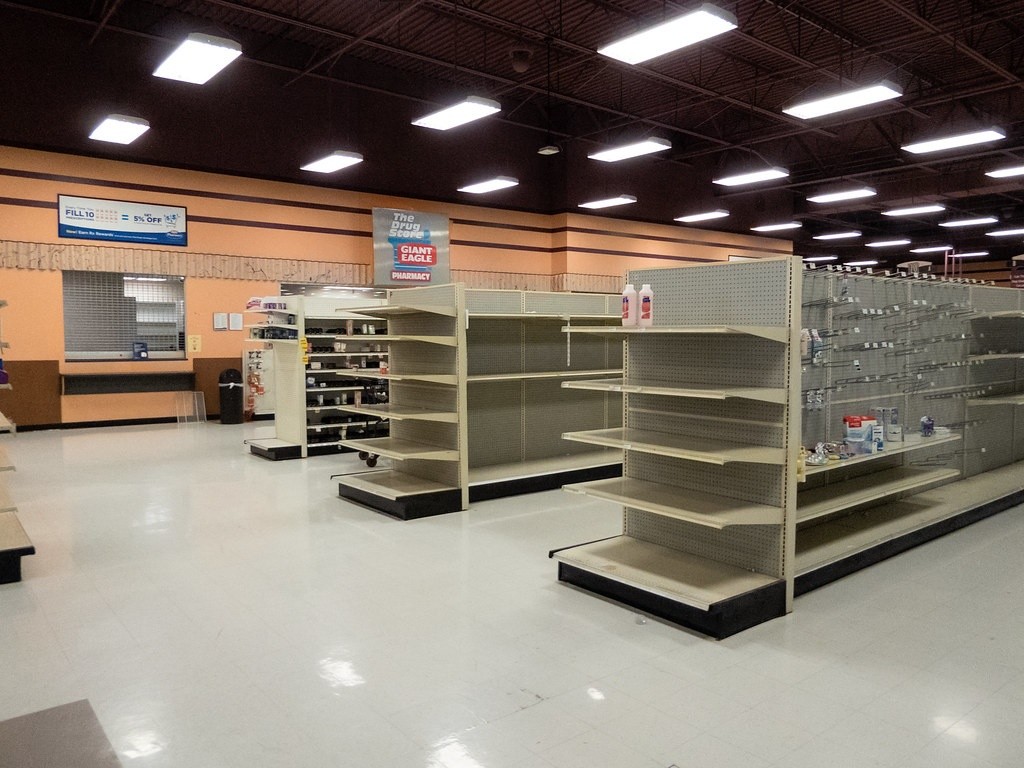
[596,2,739,70]
[674,204,733,224]
[749,216,806,234]
[508,23,534,73]
[710,163,791,189]
[802,252,841,263]
[410,92,502,132]
[298,149,365,176]
[456,163,520,194]
[900,124,1008,157]
[984,160,1024,179]
[149,29,243,85]
[841,212,1024,269]
[87,112,153,146]
[810,226,864,241]
[804,182,882,206]
[880,201,948,218]
[577,194,639,211]
[780,77,905,124]
[586,135,672,163]
[536,37,565,155]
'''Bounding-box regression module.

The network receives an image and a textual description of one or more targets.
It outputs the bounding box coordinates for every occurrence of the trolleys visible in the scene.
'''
[349,382,388,468]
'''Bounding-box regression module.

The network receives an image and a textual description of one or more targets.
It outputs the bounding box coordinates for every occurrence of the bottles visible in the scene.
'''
[638,283,653,328]
[621,284,637,327]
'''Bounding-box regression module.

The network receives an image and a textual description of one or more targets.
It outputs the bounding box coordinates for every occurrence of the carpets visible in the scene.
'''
[0,698,124,768]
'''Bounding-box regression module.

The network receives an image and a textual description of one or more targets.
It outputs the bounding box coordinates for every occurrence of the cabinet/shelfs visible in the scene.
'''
[243,296,308,461]
[334,281,469,521]
[553,254,805,641]
[305,316,390,457]
[464,287,623,504]
[794,266,1024,601]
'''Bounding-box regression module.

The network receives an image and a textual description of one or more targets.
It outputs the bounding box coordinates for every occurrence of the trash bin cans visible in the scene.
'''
[218,368,244,424]
